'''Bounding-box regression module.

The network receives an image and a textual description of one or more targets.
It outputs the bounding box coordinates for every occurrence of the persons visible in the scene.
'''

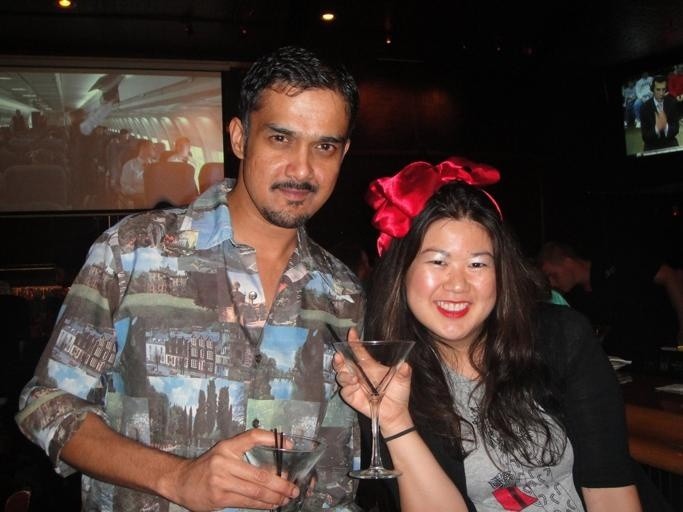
[13,47,367,512]
[537,241,683,362]
[621,64,683,151]
[333,156,642,512]
[9,109,197,210]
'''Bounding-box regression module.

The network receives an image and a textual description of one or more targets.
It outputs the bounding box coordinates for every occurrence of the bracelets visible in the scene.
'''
[381,425,418,443]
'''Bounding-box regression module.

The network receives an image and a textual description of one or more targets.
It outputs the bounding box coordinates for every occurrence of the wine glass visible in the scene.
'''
[332,339,417,480]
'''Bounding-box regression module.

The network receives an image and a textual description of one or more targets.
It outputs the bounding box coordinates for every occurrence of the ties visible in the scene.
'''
[657,102,663,112]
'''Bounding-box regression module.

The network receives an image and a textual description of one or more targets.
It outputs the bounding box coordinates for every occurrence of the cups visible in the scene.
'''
[244,430,327,512]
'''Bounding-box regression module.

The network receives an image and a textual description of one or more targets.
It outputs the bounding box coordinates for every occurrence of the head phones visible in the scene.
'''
[650,76,669,93]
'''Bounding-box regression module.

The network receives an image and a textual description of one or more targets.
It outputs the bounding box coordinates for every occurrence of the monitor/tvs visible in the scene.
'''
[606,49,683,167]
